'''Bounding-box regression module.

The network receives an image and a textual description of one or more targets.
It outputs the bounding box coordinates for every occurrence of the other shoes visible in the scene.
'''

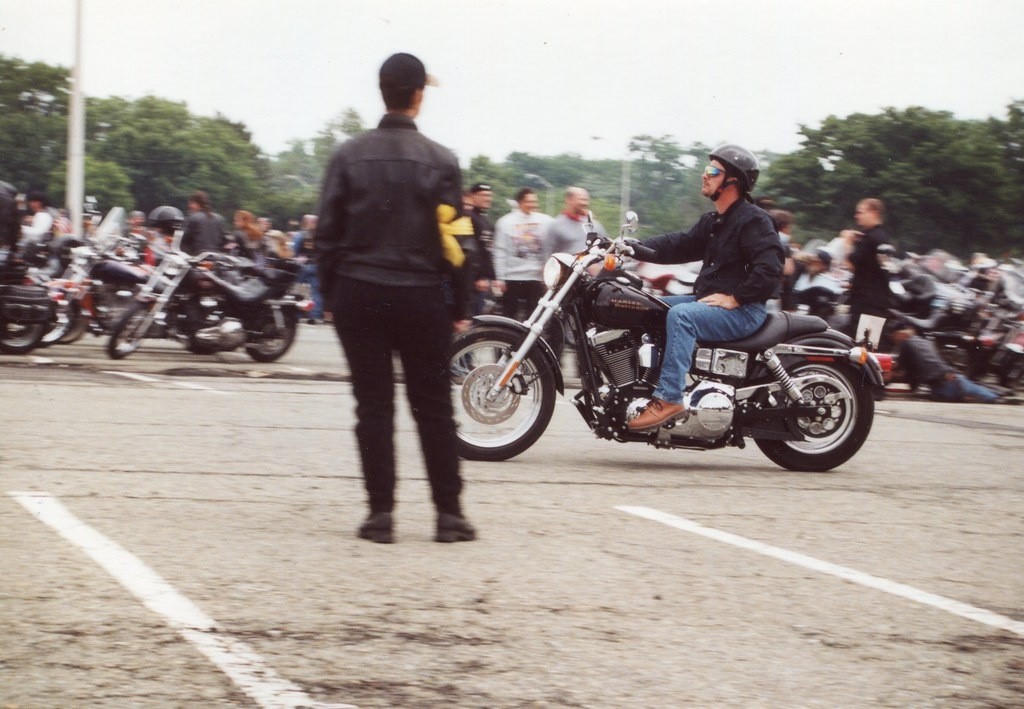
[436,512,474,541]
[361,513,393,542]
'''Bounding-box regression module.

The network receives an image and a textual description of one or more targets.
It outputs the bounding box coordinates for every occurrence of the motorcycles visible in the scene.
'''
[447,210,912,473]
[0,178,314,363]
[875,243,1024,392]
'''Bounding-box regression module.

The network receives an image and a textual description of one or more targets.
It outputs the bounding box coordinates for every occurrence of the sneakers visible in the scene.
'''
[629,397,686,432]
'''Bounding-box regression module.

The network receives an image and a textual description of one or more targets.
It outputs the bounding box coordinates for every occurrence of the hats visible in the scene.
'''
[471,183,492,192]
[380,53,439,88]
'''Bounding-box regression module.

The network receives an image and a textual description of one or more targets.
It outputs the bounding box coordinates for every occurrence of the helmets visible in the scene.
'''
[709,144,759,192]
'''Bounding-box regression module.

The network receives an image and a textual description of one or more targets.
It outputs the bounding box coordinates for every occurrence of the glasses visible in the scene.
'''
[705,164,726,177]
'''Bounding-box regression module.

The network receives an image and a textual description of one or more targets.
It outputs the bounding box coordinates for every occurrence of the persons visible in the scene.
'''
[616,144,784,431]
[0,180,324,334]
[751,196,1024,405]
[451,182,612,386]
[313,53,478,544]
